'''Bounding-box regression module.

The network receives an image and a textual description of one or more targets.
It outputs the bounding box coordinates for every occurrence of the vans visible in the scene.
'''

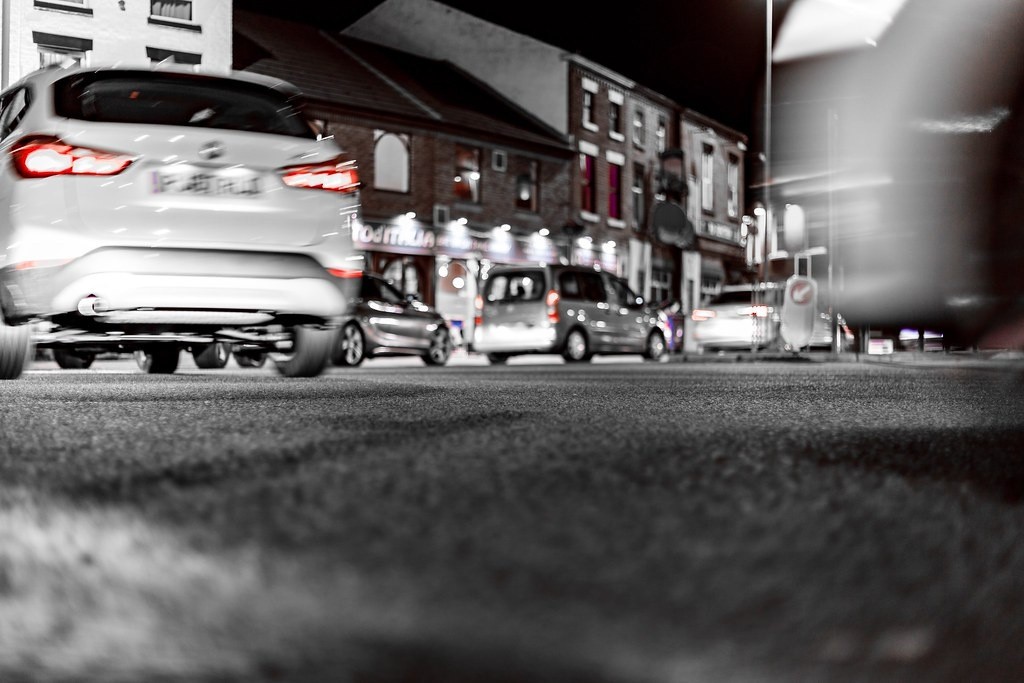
[470,263,673,366]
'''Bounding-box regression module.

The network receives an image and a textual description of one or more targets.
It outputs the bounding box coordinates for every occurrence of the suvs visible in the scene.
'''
[2,56,363,381]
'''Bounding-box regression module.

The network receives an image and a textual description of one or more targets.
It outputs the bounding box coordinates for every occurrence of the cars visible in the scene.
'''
[336,273,452,367]
[697,280,841,356]
